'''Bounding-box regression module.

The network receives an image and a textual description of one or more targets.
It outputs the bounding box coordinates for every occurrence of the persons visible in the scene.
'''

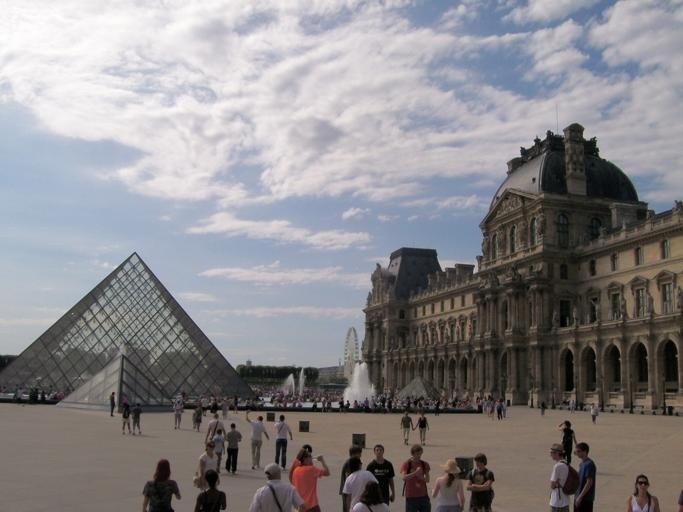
[414,411,430,445]
[245,388,507,421]
[0,383,70,403]
[399,444,431,511]
[466,453,495,511]
[549,443,569,511]
[245,410,270,470]
[558,420,577,464]
[429,459,465,511]
[273,414,293,471]
[172,389,242,475]
[200,441,221,493]
[400,411,413,445]
[626,473,659,511]
[250,463,304,511]
[678,488,683,512]
[110,392,143,435]
[541,395,598,423]
[573,442,596,511]
[142,459,181,511]
[289,444,330,511]
[340,444,395,510]
[195,469,227,511]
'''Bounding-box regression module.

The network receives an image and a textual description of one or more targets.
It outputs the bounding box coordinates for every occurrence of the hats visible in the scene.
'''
[296,447,308,465]
[440,459,461,474]
[264,462,283,478]
[548,443,565,454]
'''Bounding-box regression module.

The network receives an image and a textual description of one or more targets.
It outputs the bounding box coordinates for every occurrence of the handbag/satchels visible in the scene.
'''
[192,475,200,488]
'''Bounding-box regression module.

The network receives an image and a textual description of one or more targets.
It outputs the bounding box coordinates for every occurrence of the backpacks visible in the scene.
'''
[561,460,579,495]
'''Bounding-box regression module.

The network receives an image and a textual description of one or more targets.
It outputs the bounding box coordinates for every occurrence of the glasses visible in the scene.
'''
[638,481,647,485]
